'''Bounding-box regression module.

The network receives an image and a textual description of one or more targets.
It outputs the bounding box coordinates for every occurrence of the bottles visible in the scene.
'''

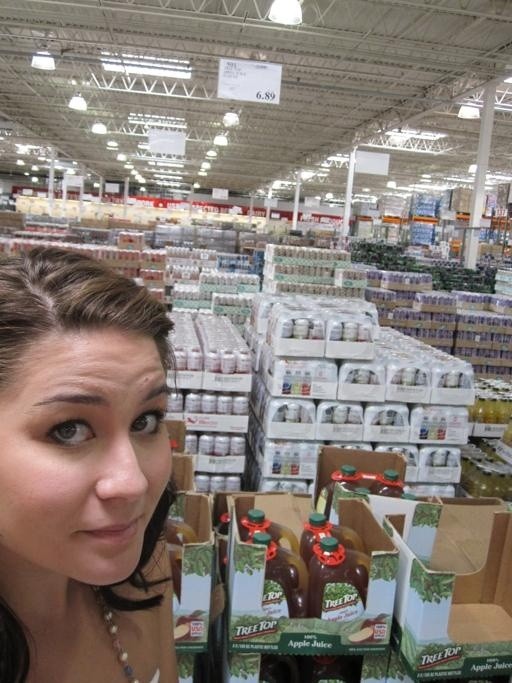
[461,375,511,502]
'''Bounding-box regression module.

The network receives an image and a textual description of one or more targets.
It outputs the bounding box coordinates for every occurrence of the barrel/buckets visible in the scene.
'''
[165,520,199,602]
[241,466,404,622]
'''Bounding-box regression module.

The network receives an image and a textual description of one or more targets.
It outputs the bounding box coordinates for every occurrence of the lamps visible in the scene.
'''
[66,93,90,112]
[265,1,306,28]
[115,154,127,162]
[30,49,57,72]
[213,135,229,147]
[204,151,218,160]
[193,162,213,189]
[139,187,146,192]
[106,141,119,152]
[457,105,480,120]
[221,110,240,127]
[468,164,479,174]
[124,163,134,169]
[130,170,146,185]
[92,121,107,135]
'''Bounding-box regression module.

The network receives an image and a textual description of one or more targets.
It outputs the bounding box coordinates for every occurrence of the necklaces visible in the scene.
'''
[93,584,138,681]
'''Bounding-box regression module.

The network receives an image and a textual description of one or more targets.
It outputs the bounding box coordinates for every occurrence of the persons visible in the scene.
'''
[0,244,179,682]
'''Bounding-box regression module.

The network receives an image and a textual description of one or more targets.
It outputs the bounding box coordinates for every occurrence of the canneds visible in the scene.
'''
[166,294,475,499]
